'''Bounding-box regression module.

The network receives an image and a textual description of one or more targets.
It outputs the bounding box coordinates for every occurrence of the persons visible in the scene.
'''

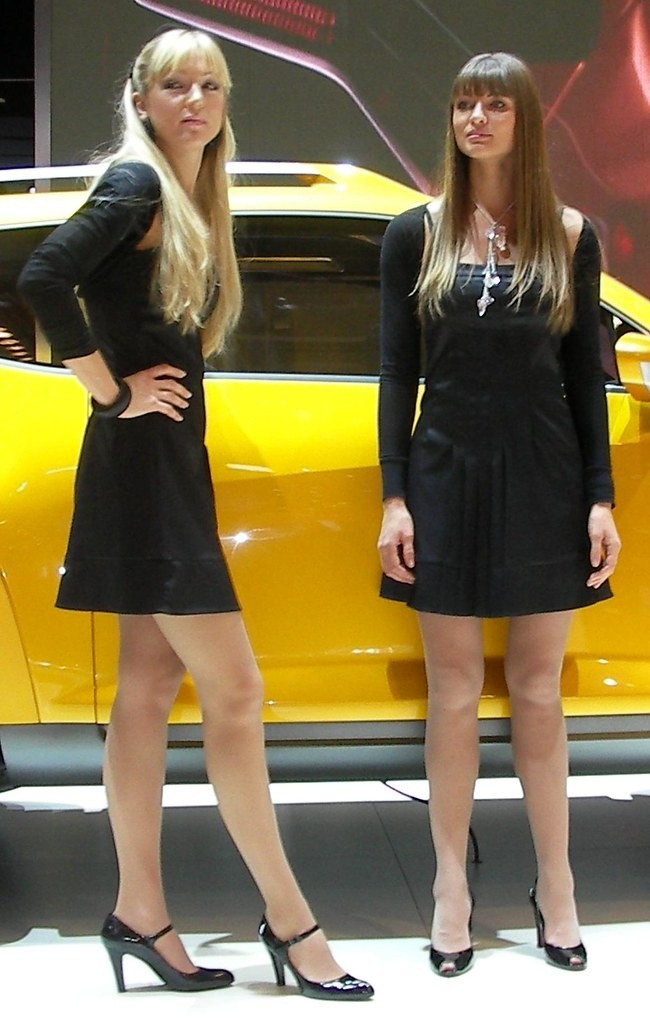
[376,51,621,977]
[20,30,374,1002]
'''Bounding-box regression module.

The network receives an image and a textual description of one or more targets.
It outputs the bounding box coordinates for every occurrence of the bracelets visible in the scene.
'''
[89,376,131,418]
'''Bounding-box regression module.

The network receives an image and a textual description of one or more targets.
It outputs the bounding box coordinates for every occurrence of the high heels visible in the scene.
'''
[429,892,475,975]
[259,915,375,1000]
[101,913,235,993]
[530,877,588,971]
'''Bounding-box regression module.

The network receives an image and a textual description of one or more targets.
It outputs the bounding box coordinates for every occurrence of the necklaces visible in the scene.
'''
[468,196,520,316]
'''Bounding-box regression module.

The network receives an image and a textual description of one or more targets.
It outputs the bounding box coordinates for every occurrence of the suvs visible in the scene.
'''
[1,161,650,793]
